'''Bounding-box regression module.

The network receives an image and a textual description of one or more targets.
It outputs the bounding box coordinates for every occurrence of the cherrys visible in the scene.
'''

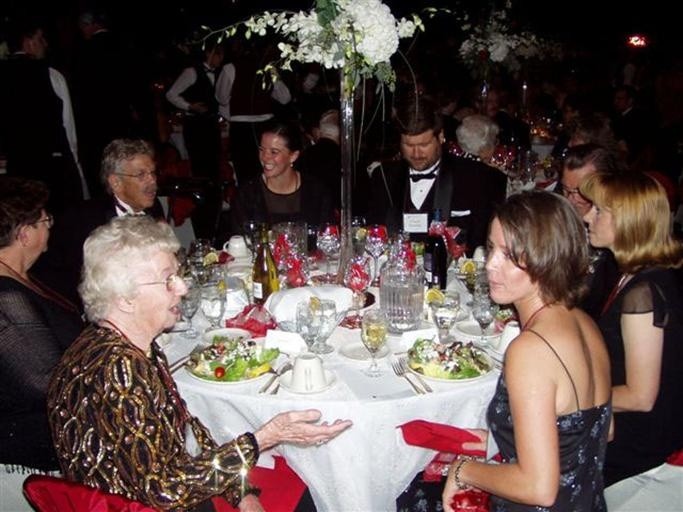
[214,367,225,379]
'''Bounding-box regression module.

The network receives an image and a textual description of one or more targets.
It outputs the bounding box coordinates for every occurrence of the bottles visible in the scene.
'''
[252,221,279,307]
[423,206,446,290]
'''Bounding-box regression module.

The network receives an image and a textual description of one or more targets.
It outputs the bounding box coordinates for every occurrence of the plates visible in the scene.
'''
[405,352,495,383]
[202,328,250,345]
[185,347,286,385]
[339,341,388,360]
[278,370,335,394]
[455,322,502,340]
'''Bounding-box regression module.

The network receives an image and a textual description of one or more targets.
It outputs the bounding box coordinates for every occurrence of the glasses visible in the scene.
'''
[110,167,160,181]
[555,184,588,205]
[14,211,53,241]
[486,138,498,148]
[137,262,185,292]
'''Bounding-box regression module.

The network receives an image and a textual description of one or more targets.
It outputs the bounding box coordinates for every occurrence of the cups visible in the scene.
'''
[275,222,306,289]
[498,320,520,353]
[292,355,325,389]
[192,237,211,260]
[222,235,247,258]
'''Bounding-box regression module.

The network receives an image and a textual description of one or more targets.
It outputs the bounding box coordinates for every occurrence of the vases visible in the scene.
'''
[327,64,364,285]
[473,76,507,117]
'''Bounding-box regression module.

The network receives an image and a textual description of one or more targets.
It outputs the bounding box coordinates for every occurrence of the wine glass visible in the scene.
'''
[315,222,340,282]
[431,289,460,342]
[366,225,387,287]
[178,286,201,340]
[360,310,386,378]
[295,300,321,359]
[343,258,370,322]
[472,278,498,346]
[200,283,226,330]
[314,300,338,354]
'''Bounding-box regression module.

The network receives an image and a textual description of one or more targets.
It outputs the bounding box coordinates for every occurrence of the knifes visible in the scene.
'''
[399,357,435,393]
[259,363,292,392]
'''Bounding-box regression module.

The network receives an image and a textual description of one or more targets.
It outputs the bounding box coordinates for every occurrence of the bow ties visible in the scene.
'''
[407,160,439,182]
[203,64,214,73]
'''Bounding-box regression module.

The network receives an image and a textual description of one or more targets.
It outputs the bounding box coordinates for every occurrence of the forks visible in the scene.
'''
[390,360,424,396]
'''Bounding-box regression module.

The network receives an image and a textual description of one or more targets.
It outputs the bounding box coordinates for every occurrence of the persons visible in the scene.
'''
[0,0,682,512]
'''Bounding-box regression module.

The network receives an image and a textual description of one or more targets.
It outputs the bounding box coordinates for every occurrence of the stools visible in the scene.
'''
[606,449,682,508]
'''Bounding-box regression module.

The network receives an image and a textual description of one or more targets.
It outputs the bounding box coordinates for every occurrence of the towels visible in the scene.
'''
[396,418,503,462]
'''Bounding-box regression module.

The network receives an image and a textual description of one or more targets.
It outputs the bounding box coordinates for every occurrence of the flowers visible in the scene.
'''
[431,1,574,99]
[200,0,428,100]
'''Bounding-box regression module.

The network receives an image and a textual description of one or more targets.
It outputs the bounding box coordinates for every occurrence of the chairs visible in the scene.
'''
[20,470,167,512]
[150,176,233,251]
